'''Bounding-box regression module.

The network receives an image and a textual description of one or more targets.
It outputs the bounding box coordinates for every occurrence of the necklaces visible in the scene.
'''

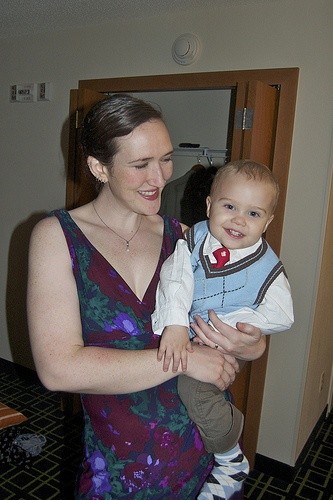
[91,200,143,247]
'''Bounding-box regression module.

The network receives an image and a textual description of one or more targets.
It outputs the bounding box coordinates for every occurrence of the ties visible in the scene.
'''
[211,248,230,269]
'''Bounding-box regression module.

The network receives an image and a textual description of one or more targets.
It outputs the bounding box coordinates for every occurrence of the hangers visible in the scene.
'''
[194,149,216,169]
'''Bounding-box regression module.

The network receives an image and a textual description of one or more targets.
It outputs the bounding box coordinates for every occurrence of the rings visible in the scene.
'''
[214,343,219,350]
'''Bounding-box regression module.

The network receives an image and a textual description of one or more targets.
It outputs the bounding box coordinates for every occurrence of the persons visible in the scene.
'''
[26,93,233,500]
[151,159,294,500]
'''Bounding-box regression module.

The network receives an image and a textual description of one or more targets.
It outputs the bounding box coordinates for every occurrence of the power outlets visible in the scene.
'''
[11,82,48,102]
